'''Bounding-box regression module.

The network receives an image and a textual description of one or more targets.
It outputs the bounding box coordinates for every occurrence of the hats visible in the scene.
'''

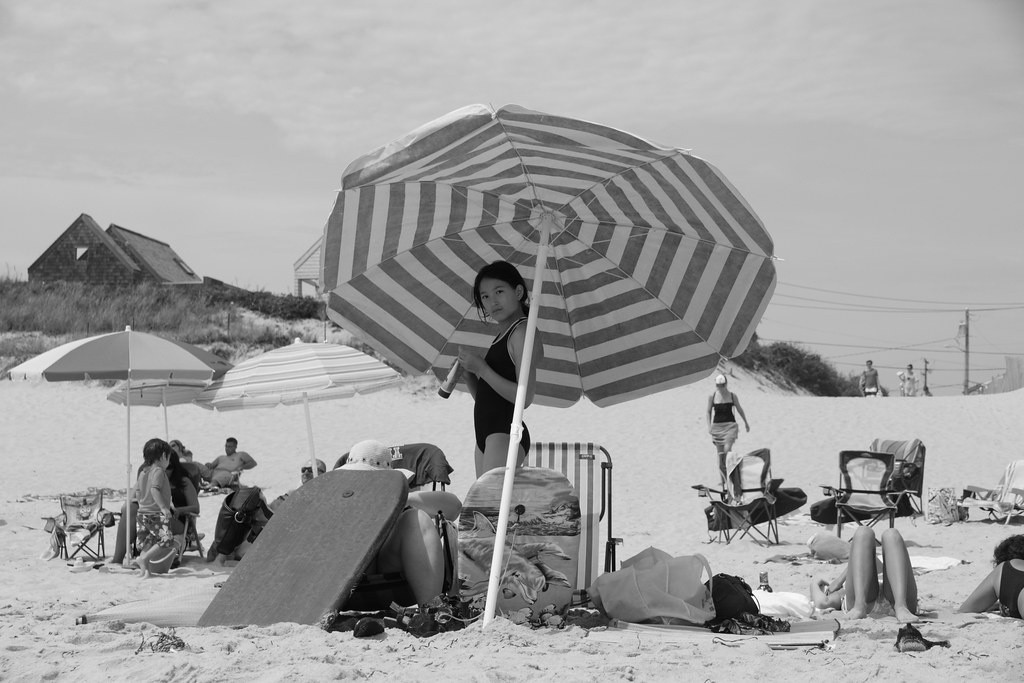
[715,374,727,385]
[334,440,415,484]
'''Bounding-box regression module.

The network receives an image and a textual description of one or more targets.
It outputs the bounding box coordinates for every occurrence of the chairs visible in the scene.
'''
[42,441,624,624]
[691,439,1023,548]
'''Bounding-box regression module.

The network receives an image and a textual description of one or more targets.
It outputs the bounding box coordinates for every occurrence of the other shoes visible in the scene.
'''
[197,533,205,540]
[353,617,385,637]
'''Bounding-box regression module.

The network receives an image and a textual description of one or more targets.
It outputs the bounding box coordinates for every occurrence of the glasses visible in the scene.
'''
[301,466,325,474]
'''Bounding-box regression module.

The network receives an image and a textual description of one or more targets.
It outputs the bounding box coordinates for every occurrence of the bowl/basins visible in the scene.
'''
[68,562,95,572]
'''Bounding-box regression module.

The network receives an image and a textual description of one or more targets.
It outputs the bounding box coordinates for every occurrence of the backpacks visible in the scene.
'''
[215,485,262,556]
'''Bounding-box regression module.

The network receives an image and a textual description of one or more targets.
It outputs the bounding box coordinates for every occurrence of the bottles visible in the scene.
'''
[757,572,772,593]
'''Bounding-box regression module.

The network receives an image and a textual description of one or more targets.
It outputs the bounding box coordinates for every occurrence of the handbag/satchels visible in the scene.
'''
[584,548,760,625]
[928,487,963,524]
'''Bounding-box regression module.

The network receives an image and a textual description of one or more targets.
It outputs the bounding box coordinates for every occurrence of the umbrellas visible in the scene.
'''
[106,377,208,443]
[193,336,406,479]
[5,326,233,567]
[321,103,778,634]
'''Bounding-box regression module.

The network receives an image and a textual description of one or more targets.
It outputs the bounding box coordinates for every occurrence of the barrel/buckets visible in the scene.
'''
[149,539,180,573]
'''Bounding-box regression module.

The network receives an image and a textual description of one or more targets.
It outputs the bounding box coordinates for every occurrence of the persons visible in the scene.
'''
[457,260,544,478]
[268,438,463,608]
[707,375,749,485]
[111,436,257,577]
[809,526,921,622]
[958,535,1024,619]
[898,364,918,396]
[862,360,880,398]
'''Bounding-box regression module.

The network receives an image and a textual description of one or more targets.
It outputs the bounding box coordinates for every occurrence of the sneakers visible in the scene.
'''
[895,623,927,652]
